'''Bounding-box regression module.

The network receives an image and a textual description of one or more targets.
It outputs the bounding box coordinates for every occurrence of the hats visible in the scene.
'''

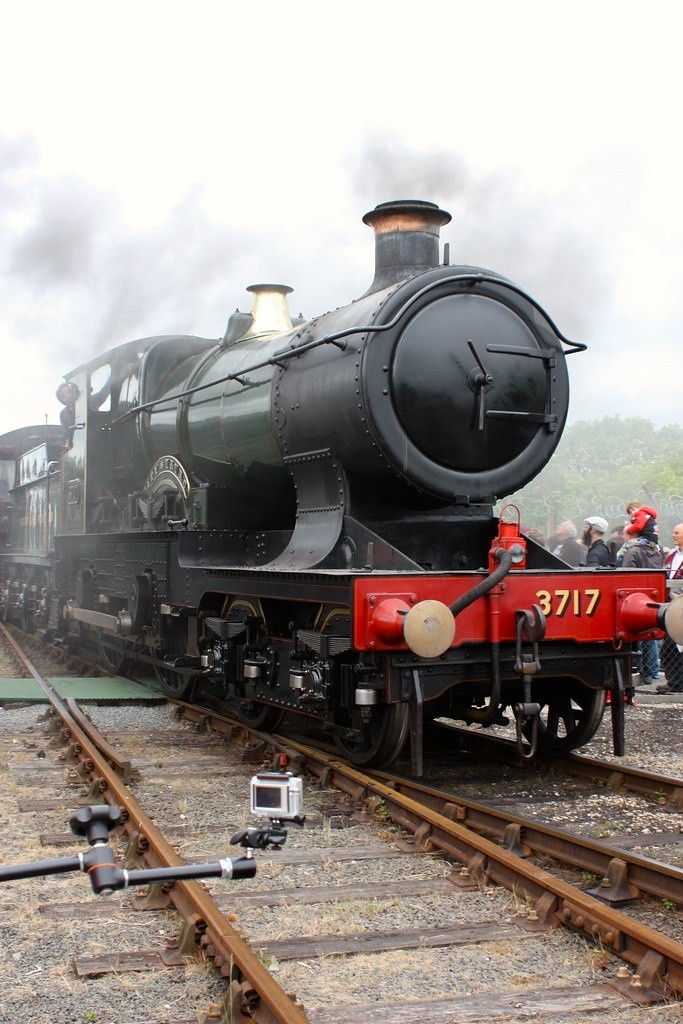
[584,516,608,533]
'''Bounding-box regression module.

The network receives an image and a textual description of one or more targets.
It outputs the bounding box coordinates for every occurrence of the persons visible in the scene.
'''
[519,502,683,705]
[57,384,78,439]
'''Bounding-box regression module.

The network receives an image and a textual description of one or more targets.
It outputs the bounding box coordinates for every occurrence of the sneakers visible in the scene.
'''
[639,669,659,683]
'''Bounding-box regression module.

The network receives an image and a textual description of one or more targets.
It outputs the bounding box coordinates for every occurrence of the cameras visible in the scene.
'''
[250,777,303,817]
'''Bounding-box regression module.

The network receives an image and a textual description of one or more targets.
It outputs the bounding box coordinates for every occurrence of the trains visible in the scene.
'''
[3,195,683,771]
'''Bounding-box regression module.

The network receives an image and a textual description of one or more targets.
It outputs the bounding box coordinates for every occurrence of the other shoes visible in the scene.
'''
[656,684,683,691]
[611,561,621,566]
